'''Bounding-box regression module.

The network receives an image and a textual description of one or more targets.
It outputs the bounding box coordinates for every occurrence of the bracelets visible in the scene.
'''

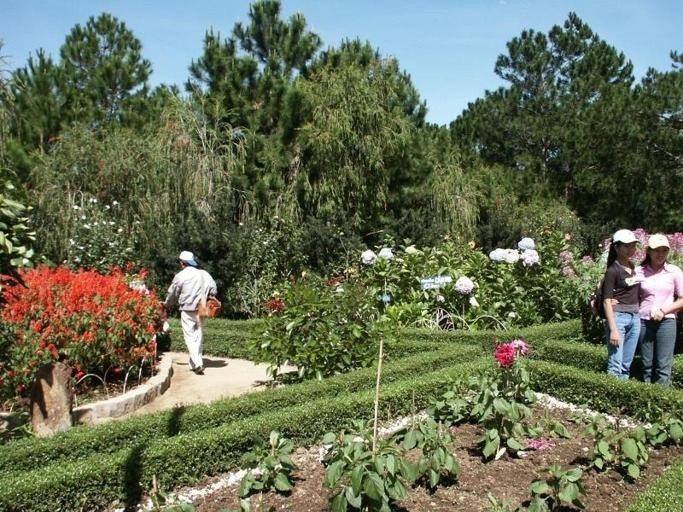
[659,308,666,316]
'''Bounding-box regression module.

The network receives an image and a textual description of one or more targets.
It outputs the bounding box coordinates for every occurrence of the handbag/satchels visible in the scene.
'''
[595,280,612,318]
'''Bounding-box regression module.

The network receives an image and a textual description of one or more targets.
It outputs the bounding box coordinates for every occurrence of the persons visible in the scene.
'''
[129,265,149,296]
[602,229,641,381]
[162,250,218,374]
[635,234,683,385]
[157,311,171,351]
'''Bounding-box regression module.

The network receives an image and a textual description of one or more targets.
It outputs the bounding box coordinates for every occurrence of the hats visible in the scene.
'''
[613,229,641,244]
[177,250,198,267]
[647,234,670,250]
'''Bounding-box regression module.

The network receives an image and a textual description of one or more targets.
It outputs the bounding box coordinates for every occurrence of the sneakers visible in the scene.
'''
[194,366,205,374]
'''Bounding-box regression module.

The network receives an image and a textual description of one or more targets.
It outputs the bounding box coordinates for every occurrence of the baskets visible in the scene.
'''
[198,296,222,318]
